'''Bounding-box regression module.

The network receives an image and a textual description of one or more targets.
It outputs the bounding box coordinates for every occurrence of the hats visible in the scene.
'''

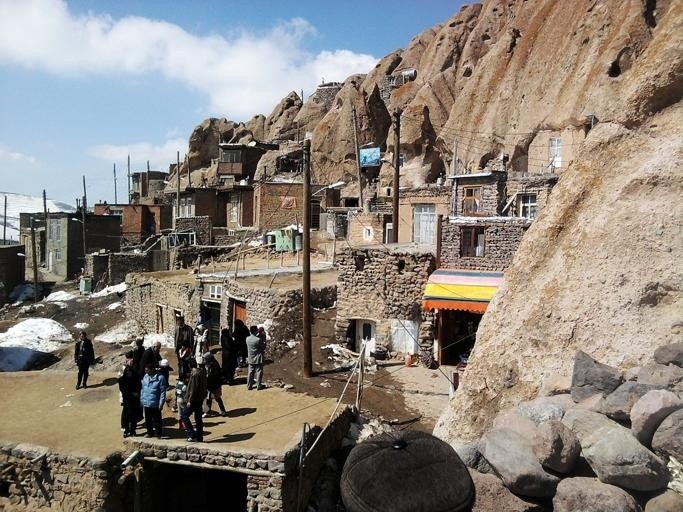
[160,359,169,367]
[338,428,476,512]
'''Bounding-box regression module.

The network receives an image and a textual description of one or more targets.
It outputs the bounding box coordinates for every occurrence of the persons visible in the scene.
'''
[73,331,95,390]
[118,316,267,442]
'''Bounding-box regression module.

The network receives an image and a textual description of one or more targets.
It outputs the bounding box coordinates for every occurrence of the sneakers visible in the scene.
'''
[202,413,210,418]
